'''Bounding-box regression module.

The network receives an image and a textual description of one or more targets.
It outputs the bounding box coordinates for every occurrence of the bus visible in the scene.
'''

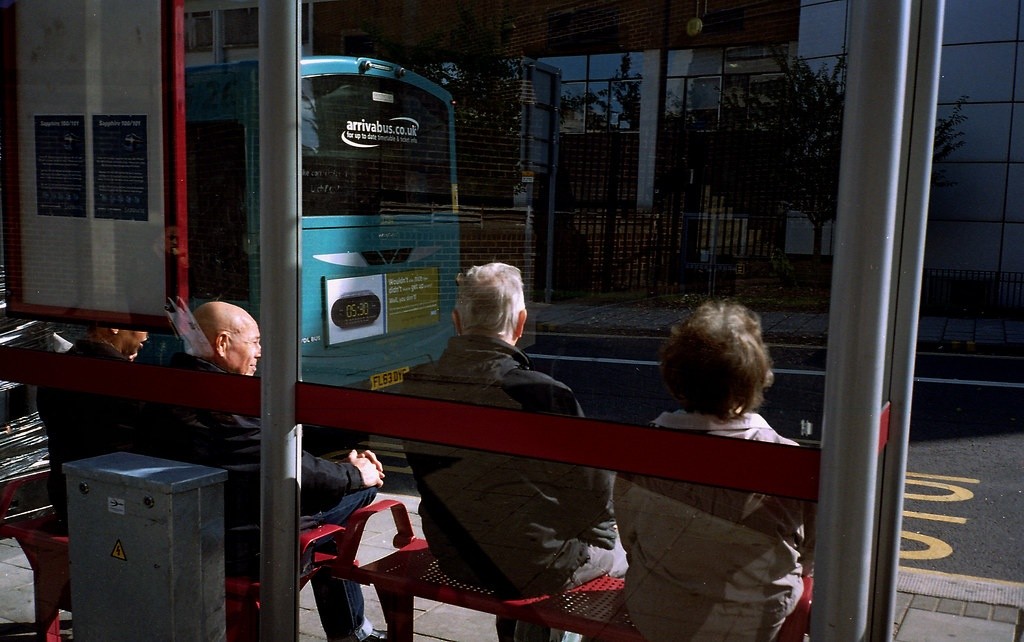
[78,55,465,368]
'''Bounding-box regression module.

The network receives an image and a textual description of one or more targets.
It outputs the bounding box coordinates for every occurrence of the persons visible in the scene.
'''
[37,324,148,525]
[125,301,388,642]
[613,302,807,642]
[402,262,617,642]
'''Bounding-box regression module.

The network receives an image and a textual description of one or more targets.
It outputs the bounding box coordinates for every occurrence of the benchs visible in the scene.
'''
[328,500,815,642]
[0,469,358,642]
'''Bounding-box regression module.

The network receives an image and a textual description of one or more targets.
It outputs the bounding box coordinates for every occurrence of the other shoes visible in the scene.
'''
[364,628,389,642]
